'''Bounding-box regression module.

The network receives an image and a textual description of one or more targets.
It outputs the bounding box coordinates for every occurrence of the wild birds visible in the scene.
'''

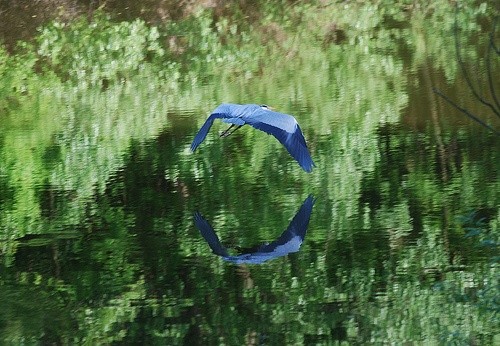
[190,103,317,172]
[193,193,319,266]
